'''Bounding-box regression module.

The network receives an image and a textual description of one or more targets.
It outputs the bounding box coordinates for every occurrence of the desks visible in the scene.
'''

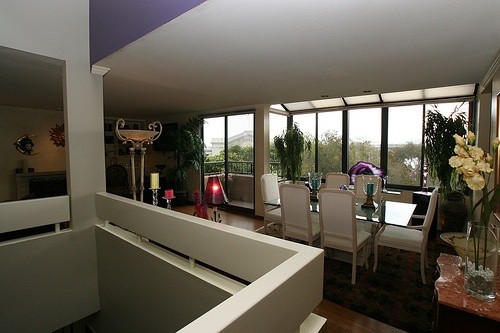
[432,250,500,333]
[264,193,418,266]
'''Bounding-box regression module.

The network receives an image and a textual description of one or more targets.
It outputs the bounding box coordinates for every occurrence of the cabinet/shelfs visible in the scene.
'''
[105,117,146,168]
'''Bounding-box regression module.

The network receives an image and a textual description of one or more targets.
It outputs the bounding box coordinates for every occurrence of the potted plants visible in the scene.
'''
[426,113,468,231]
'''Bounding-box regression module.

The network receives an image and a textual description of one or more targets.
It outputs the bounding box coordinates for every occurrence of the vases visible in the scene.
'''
[466,220,500,298]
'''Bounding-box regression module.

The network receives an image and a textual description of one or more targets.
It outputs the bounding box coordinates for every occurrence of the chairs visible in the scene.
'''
[325,173,350,190]
[316,188,371,284]
[279,183,320,246]
[260,172,281,234]
[373,187,438,284]
[354,175,382,199]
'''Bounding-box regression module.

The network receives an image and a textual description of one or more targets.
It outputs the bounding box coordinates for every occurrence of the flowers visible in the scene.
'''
[449,132,500,223]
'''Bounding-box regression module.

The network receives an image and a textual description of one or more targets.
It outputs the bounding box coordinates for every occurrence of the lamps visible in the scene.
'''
[205,176,230,222]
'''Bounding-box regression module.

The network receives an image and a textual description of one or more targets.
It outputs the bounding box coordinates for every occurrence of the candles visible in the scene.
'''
[150,172,159,188]
[164,189,173,197]
